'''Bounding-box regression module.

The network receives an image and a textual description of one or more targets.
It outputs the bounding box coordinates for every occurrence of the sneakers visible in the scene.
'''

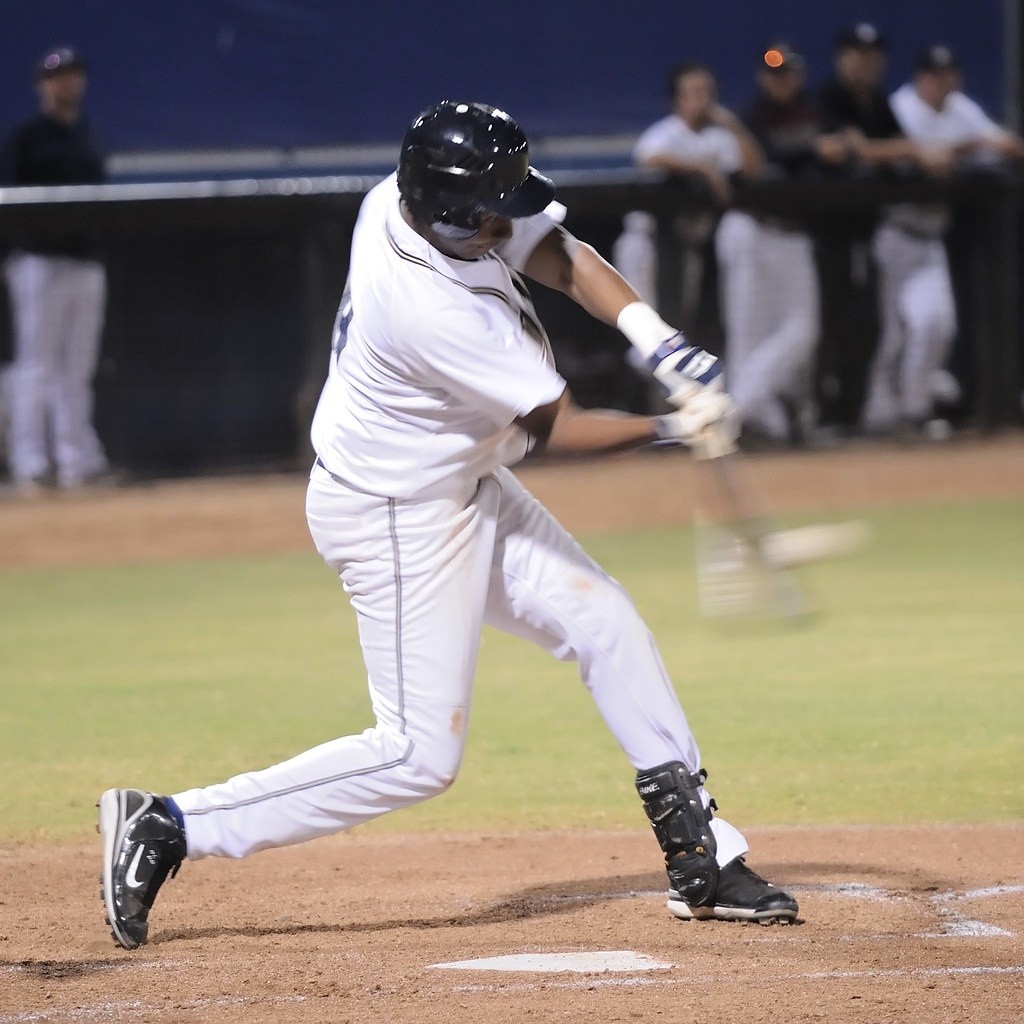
[97,788,188,951]
[667,856,798,925]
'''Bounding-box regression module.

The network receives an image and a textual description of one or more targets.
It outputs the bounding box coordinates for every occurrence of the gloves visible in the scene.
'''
[655,392,740,462]
[619,302,726,406]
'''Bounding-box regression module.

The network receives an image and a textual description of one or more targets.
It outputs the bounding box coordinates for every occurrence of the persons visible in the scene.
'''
[96,101,800,950]
[2,23,1024,493]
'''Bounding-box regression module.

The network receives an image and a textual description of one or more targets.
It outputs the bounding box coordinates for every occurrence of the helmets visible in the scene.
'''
[397,99,556,240]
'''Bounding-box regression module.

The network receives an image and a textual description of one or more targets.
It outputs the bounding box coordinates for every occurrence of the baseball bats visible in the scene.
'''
[655,370,815,628]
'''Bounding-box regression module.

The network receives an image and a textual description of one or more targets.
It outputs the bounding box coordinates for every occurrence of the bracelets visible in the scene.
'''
[616,300,679,364]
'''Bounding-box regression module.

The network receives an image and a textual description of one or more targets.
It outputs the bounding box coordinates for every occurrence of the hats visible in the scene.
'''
[912,43,964,73]
[832,16,891,51]
[29,40,84,83]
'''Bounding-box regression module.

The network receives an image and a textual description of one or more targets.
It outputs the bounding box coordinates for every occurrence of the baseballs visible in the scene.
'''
[762,521,851,566]
